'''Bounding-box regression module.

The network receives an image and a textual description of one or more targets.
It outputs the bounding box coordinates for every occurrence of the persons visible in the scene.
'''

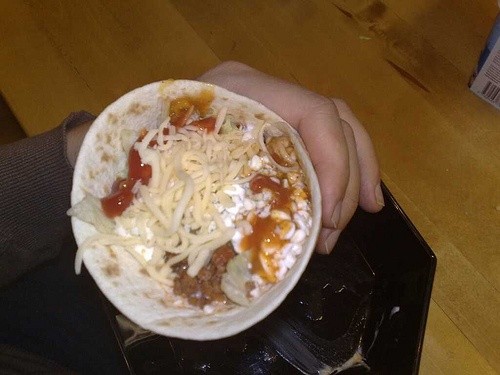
[0,62,384,374]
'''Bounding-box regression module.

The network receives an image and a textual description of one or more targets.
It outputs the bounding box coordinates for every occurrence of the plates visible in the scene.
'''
[61,75,321,344]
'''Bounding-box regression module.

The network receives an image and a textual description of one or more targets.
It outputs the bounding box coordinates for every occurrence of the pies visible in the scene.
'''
[67,77,322,343]
